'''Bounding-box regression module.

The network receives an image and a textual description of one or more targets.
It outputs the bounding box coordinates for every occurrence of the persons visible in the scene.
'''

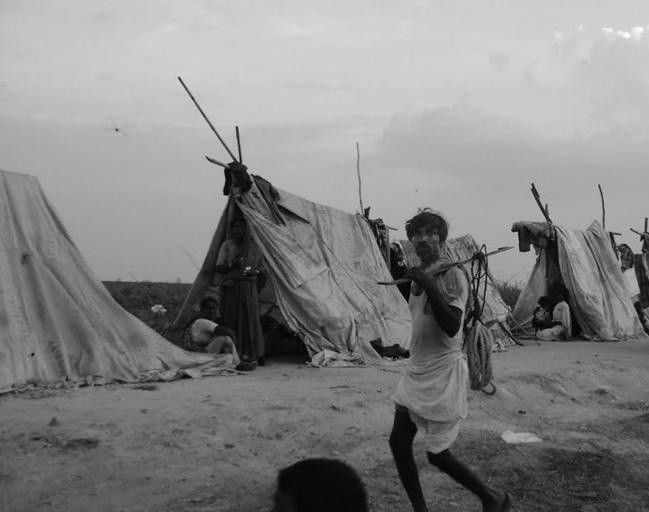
[215,214,268,367]
[267,455,368,512]
[551,287,572,340]
[531,295,562,340]
[181,297,257,374]
[388,210,517,510]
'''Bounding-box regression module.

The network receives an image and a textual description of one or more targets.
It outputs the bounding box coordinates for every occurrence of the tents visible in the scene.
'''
[506,218,645,342]
[0,166,234,397]
[392,232,509,334]
[162,156,413,370]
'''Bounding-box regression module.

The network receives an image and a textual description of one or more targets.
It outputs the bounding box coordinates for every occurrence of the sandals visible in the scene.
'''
[482,487,513,512]
